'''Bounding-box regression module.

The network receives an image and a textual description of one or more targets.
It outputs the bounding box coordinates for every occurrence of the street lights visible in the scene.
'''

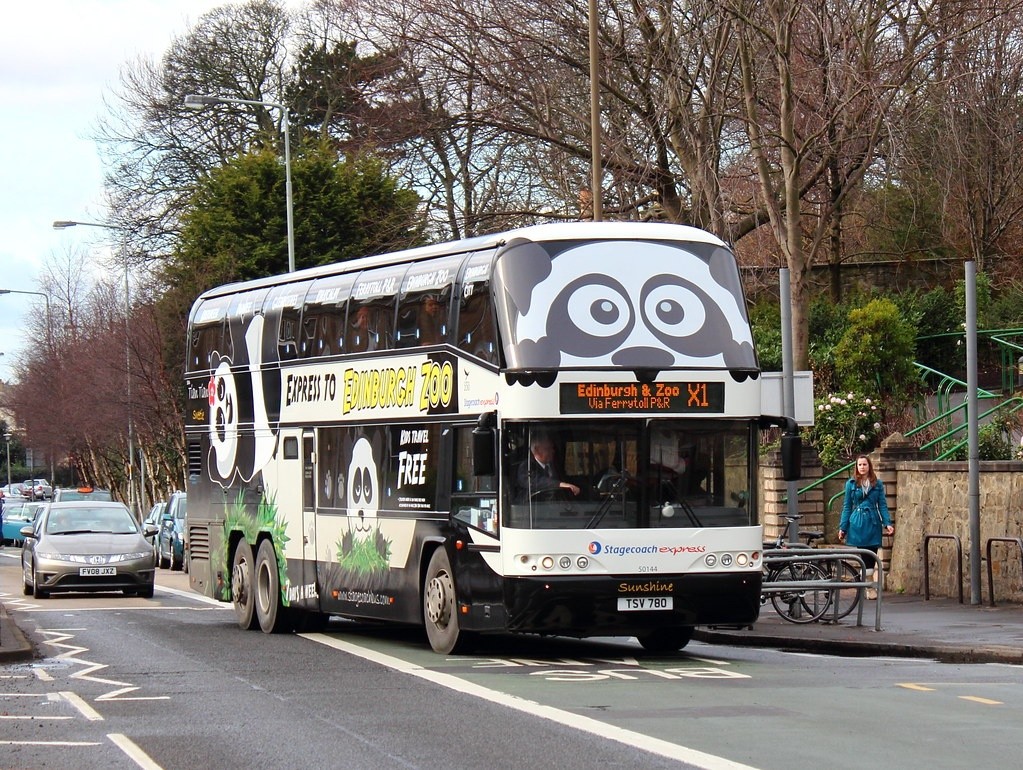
[185,94,296,272]
[3,433,14,494]
[0,289,52,363]
[53,220,140,519]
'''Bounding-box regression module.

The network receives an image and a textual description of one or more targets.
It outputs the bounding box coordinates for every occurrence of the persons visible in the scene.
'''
[518,433,581,503]
[839,454,894,600]
[58,513,70,530]
[0,490,7,549]
[419,293,440,345]
[352,309,371,352]
[648,426,689,497]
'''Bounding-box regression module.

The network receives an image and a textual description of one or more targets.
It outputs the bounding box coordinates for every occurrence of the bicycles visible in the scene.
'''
[767,514,862,626]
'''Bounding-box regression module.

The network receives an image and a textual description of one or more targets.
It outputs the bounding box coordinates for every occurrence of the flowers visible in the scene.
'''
[802,387,880,470]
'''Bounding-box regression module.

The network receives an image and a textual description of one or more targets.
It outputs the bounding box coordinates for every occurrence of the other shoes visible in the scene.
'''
[0,545,5,550]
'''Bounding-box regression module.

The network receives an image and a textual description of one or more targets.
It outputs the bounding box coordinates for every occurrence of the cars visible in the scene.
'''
[1,479,116,546]
[19,500,158,597]
[141,503,168,565]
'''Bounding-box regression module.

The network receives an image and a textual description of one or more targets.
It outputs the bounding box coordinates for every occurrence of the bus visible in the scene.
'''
[185,222,803,657]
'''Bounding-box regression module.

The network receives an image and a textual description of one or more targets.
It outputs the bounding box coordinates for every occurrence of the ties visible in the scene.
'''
[545,465,549,478]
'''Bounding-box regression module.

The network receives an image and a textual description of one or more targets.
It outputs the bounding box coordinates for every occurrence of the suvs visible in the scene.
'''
[154,490,189,570]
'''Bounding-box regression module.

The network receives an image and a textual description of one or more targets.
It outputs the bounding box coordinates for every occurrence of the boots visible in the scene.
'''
[860,569,877,599]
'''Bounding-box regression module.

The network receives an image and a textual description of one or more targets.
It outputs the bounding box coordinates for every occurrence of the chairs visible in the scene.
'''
[286,325,495,365]
[194,353,212,371]
